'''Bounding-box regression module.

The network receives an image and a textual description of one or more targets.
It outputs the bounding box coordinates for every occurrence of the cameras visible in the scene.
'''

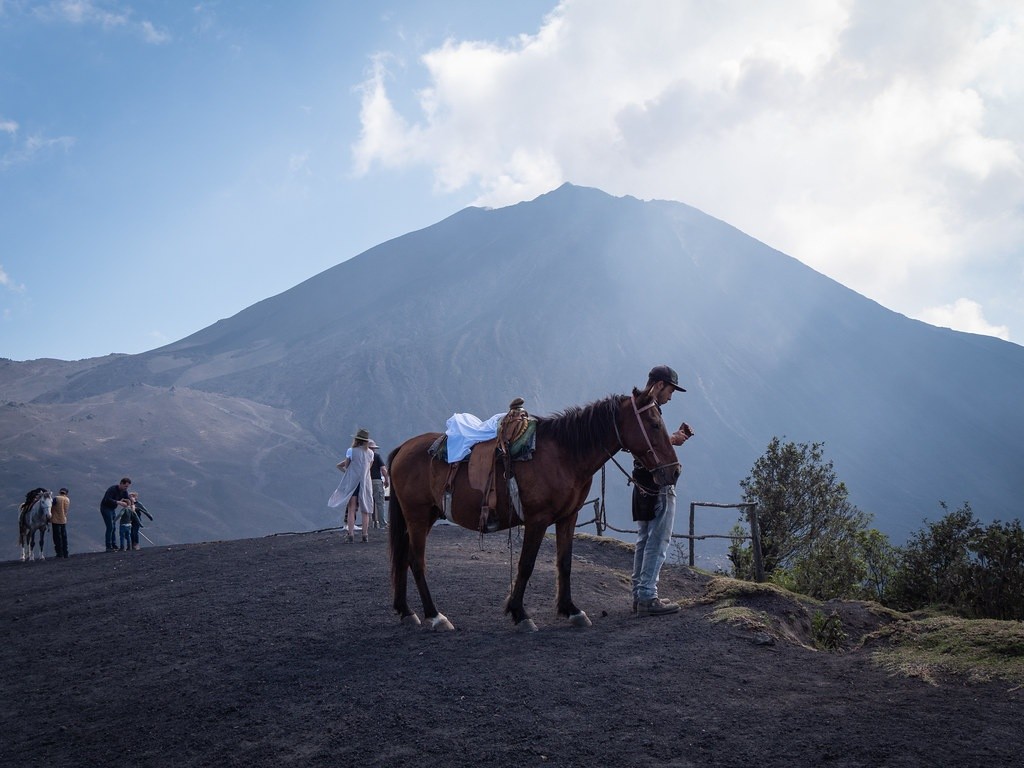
[680,423,694,439]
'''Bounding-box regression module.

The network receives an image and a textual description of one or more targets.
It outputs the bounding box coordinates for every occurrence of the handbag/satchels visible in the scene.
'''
[632,470,658,521]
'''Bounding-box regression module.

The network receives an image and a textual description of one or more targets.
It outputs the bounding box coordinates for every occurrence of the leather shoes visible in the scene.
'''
[637,599,681,616]
[632,597,670,612]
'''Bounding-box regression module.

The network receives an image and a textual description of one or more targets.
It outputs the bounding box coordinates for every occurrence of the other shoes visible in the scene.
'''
[127,546,132,550]
[106,548,114,552]
[132,544,140,551]
[113,546,119,551]
[119,548,126,551]
[344,534,354,544]
[360,535,368,544]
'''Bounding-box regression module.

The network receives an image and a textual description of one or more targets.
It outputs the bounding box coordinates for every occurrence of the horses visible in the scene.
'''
[19,490,54,561]
[387,380,682,635]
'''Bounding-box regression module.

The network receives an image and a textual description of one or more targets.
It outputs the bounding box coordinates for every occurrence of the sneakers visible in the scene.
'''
[343,523,362,531]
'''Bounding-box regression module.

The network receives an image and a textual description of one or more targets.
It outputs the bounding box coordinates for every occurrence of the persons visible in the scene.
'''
[49,488,70,558]
[100,478,132,552]
[629,365,690,617]
[113,498,144,552]
[337,460,363,530]
[368,439,391,529]
[344,429,375,544]
[381,466,391,528]
[129,493,153,550]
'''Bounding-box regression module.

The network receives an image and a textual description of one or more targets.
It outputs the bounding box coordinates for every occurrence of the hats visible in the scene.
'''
[367,438,379,449]
[59,488,68,494]
[650,365,686,392]
[352,428,369,441]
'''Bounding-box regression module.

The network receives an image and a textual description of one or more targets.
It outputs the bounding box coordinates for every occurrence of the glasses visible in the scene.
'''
[124,485,129,488]
